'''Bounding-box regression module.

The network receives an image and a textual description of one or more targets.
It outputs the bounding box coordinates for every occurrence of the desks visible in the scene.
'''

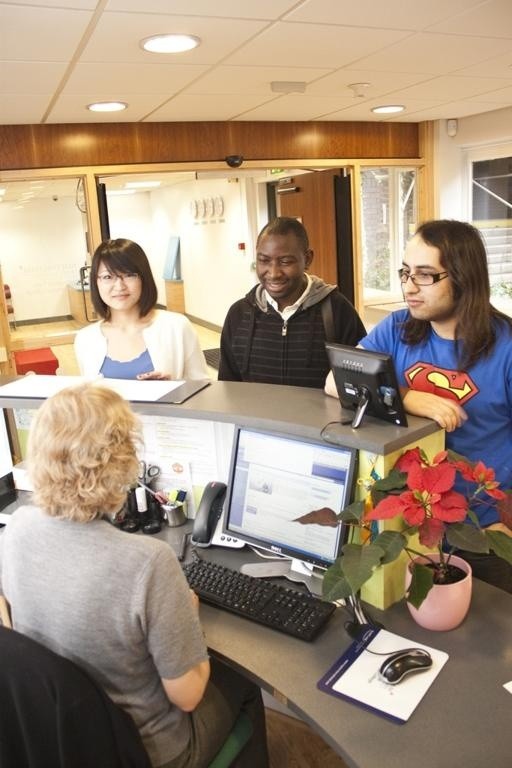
[1,489,512,768]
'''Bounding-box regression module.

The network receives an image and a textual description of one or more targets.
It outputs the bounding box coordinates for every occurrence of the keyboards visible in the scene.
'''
[181,559,336,644]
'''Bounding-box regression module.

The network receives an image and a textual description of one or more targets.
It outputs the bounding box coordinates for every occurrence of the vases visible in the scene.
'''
[405,554,471,630]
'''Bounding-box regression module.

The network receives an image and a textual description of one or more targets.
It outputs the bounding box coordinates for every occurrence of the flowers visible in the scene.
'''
[291,447,512,612]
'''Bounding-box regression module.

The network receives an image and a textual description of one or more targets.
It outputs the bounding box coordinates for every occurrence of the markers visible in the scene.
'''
[165,487,188,506]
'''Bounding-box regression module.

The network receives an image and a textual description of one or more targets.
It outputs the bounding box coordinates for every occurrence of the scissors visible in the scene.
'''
[138,461,159,483]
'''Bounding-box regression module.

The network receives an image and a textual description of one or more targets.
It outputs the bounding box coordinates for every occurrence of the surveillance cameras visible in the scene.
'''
[225,154,244,168]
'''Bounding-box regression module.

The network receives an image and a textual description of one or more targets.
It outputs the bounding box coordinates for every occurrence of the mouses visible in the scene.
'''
[379,644,431,683]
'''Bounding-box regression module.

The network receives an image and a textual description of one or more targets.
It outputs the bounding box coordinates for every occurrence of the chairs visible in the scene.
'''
[1,627,255,767]
[4,284,16,331]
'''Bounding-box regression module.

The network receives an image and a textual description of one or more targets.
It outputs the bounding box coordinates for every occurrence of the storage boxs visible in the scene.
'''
[14,348,59,375]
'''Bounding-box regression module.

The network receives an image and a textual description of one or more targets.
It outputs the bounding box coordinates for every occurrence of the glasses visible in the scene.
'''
[399,269,448,286]
[97,272,138,284]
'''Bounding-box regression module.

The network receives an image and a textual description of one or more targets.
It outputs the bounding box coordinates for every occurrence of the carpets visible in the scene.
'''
[204,348,221,370]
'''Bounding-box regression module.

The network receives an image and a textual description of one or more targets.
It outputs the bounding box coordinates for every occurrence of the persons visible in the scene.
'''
[218,216,366,391]
[74,239,210,383]
[0,387,269,766]
[324,219,512,597]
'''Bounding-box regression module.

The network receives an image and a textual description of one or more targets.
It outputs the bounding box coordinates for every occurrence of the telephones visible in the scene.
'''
[191,482,246,548]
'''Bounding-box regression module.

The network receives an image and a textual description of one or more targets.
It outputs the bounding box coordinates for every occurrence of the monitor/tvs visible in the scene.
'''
[325,340,408,433]
[220,422,360,599]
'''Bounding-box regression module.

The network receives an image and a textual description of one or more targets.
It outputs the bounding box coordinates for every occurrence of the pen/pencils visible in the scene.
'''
[177,534,188,561]
